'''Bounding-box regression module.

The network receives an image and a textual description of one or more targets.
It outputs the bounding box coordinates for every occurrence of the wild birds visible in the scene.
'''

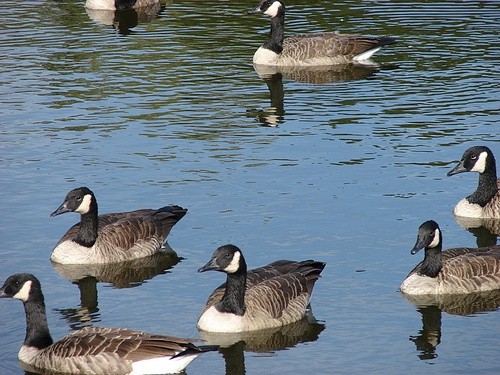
[0,273,220,375]
[245,0,400,65]
[48,187,189,266]
[446,146,500,219]
[197,244,327,333]
[399,220,500,296]
[85,0,159,12]
